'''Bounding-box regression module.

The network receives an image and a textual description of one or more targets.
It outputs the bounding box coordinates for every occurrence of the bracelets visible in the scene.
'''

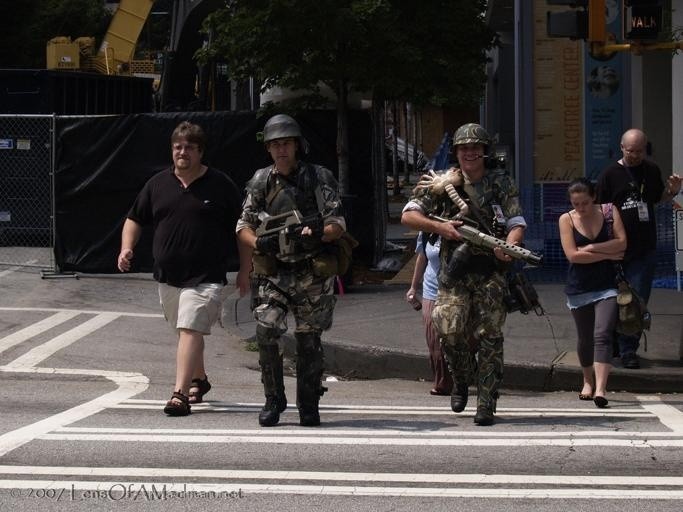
[668,189,678,196]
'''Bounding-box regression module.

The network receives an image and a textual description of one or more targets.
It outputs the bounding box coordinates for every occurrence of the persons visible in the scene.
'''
[402,122,528,425]
[559,177,627,406]
[597,129,683,369]
[405,230,452,396]
[233,113,345,421]
[117,121,253,415]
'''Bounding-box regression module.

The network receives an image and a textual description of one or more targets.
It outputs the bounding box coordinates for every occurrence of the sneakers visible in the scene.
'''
[297,404,321,427]
[621,353,641,369]
[259,393,288,427]
[450,377,469,413]
[473,404,496,426]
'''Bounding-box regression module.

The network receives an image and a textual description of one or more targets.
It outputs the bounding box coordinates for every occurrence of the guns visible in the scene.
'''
[254,212,323,250]
[428,213,545,267]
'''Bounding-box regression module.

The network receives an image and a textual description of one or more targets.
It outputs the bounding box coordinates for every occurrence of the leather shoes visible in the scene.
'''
[593,396,608,407]
[579,385,596,400]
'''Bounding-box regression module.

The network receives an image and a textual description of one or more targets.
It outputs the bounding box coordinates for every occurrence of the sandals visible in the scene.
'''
[164,389,192,416]
[187,374,212,404]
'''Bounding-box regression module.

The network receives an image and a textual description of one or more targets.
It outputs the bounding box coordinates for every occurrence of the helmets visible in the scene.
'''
[262,114,303,143]
[452,122,491,149]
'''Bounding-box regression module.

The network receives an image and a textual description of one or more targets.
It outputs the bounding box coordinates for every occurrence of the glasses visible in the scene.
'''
[172,145,199,152]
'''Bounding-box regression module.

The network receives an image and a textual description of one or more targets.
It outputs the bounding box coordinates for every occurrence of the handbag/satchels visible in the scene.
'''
[616,280,652,336]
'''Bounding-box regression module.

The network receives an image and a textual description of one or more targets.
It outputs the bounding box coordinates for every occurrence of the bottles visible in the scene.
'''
[408,294,422,311]
